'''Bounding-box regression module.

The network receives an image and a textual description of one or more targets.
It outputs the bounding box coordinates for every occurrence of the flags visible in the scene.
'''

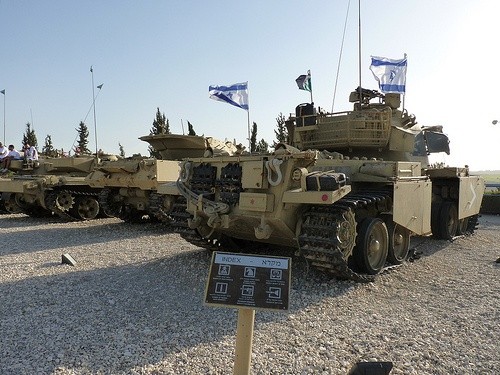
[369,55,407,95]
[209,81,249,112]
[295,73,312,92]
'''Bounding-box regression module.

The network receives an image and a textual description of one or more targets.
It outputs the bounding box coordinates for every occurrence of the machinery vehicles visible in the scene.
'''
[175,86,485,283]
[0,149,127,222]
[86,134,244,226]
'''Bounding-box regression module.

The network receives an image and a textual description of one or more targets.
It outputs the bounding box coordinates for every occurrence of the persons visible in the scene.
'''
[0,145,21,173]
[24,143,39,167]
[0,142,8,162]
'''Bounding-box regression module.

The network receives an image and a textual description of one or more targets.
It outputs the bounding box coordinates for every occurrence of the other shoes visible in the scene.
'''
[0,168,5,172]
[2,168,9,173]
